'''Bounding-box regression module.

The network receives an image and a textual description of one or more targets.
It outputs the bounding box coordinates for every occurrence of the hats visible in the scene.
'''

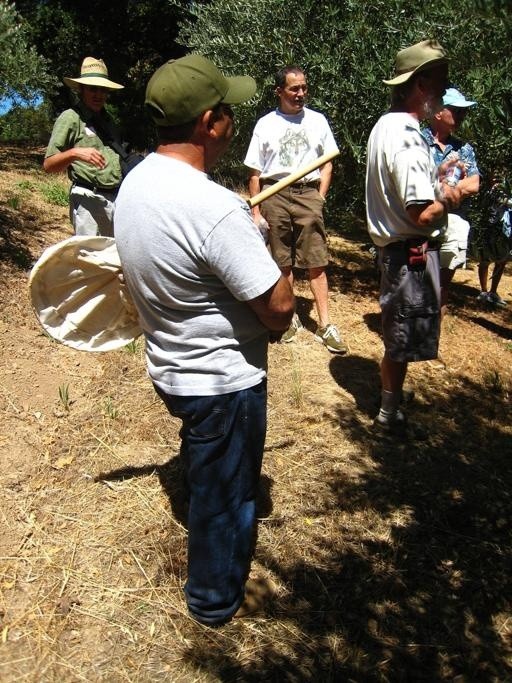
[64,56,124,90]
[145,54,257,126]
[382,39,449,86]
[443,87,477,109]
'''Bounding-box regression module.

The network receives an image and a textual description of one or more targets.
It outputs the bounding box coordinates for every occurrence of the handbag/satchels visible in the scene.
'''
[119,152,143,178]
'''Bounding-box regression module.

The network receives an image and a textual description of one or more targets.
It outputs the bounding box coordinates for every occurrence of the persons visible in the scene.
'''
[477,182,512,306]
[420,87,481,322]
[366,39,466,442]
[43,57,123,237]
[113,55,295,626]
[243,64,349,353]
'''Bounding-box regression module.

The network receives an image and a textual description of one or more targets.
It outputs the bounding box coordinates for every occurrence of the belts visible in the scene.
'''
[74,182,119,193]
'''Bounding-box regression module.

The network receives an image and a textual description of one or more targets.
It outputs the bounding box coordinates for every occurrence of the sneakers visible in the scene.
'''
[278,315,303,342]
[369,389,414,428]
[314,323,348,354]
[427,358,445,370]
[232,578,276,616]
[477,291,507,306]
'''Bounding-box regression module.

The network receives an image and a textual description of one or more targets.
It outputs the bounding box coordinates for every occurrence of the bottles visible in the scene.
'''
[442,159,463,189]
